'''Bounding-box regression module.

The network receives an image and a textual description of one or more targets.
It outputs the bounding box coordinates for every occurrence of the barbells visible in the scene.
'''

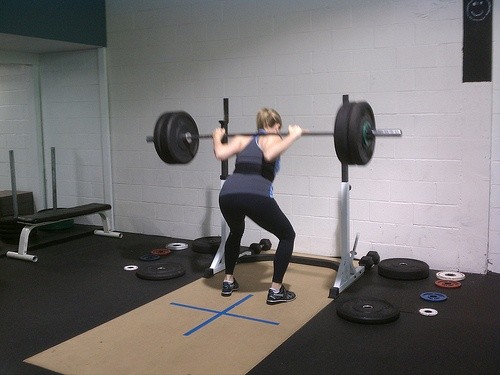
[145,101,402,165]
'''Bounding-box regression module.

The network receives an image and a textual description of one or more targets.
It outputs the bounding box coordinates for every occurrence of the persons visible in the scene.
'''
[211,107,304,305]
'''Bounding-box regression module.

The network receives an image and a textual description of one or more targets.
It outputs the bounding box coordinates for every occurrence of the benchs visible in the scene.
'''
[6,203,123,262]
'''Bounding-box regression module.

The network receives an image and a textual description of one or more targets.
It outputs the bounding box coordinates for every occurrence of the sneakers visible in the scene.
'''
[222,277,240,296]
[267,285,297,305]
